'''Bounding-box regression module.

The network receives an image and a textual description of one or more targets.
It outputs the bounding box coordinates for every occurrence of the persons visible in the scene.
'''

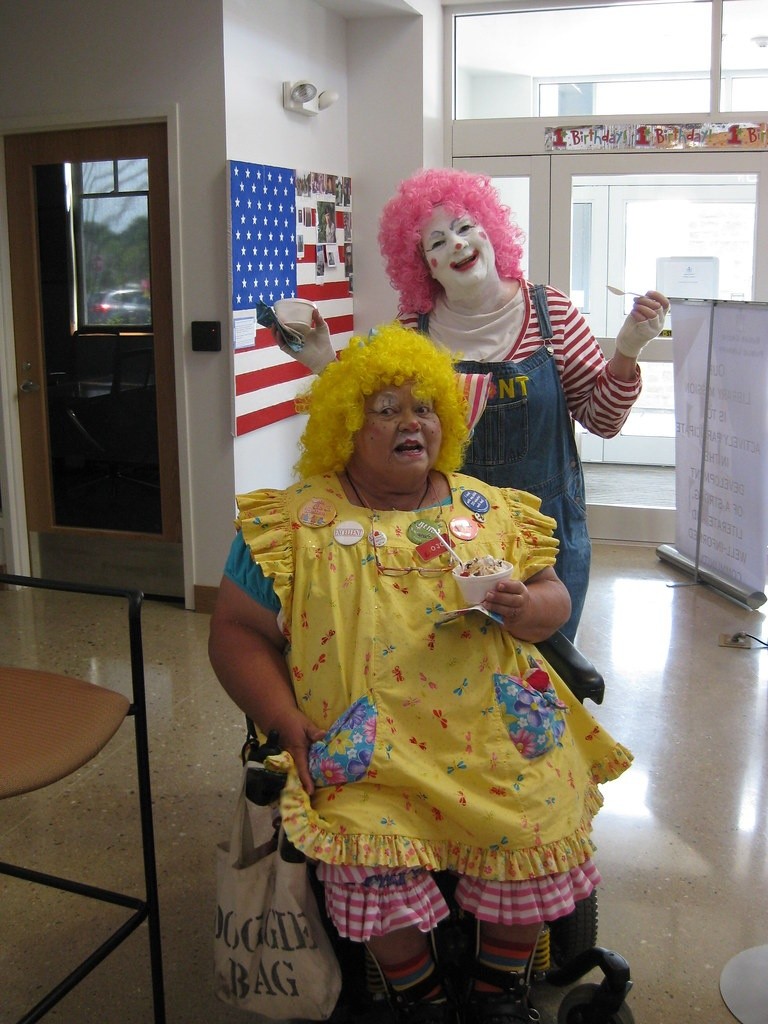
[264,165,673,647]
[207,321,634,1024]
[294,172,353,293]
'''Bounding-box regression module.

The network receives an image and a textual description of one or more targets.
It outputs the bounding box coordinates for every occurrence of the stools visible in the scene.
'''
[0,564,168,1024]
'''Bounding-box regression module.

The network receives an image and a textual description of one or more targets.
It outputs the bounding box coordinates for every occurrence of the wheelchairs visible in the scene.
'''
[233,626,639,1024]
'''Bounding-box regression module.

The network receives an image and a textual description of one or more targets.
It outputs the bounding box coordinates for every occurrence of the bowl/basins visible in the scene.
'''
[272,298,319,334]
[452,558,514,606]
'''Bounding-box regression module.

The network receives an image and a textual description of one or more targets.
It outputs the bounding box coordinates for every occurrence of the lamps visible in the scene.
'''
[282,77,339,118]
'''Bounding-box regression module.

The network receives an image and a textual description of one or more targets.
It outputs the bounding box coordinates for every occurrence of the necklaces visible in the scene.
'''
[344,468,430,511]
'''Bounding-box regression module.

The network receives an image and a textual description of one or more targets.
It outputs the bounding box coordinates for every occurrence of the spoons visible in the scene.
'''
[607,285,651,298]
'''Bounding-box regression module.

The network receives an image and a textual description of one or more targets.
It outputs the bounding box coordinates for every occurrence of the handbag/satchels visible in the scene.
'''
[212,761,342,1019]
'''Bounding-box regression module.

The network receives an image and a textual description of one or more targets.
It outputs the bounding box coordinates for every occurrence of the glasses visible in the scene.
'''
[372,517,455,579]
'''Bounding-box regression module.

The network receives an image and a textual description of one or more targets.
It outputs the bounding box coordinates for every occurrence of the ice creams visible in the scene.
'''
[459,554,506,576]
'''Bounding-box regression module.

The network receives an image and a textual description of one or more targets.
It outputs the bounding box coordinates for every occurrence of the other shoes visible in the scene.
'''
[460,946,540,1024]
[388,987,457,1024]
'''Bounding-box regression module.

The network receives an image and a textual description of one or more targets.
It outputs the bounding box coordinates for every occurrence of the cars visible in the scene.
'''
[88,288,150,322]
